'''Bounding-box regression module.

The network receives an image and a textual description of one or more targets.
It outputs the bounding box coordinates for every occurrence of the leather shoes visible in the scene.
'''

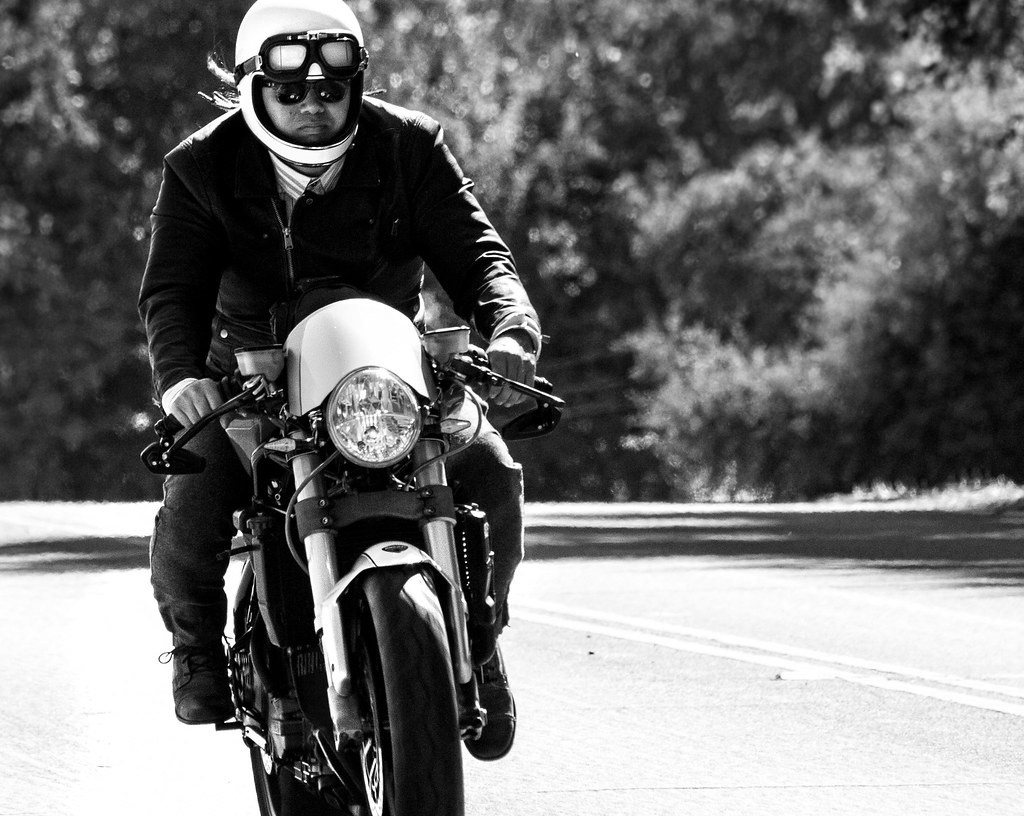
[172,642,236,725]
[452,642,517,762]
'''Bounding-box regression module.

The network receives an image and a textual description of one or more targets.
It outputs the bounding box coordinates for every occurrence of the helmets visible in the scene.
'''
[232,0,366,164]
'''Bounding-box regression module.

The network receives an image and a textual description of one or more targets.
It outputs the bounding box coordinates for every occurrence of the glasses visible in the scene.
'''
[257,80,351,106]
[233,27,371,85]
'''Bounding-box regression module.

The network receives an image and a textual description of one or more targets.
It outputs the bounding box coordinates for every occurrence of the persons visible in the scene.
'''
[136,0,545,757]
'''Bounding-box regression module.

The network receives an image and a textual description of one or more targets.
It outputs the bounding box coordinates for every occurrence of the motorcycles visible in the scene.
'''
[136,276,565,816]
